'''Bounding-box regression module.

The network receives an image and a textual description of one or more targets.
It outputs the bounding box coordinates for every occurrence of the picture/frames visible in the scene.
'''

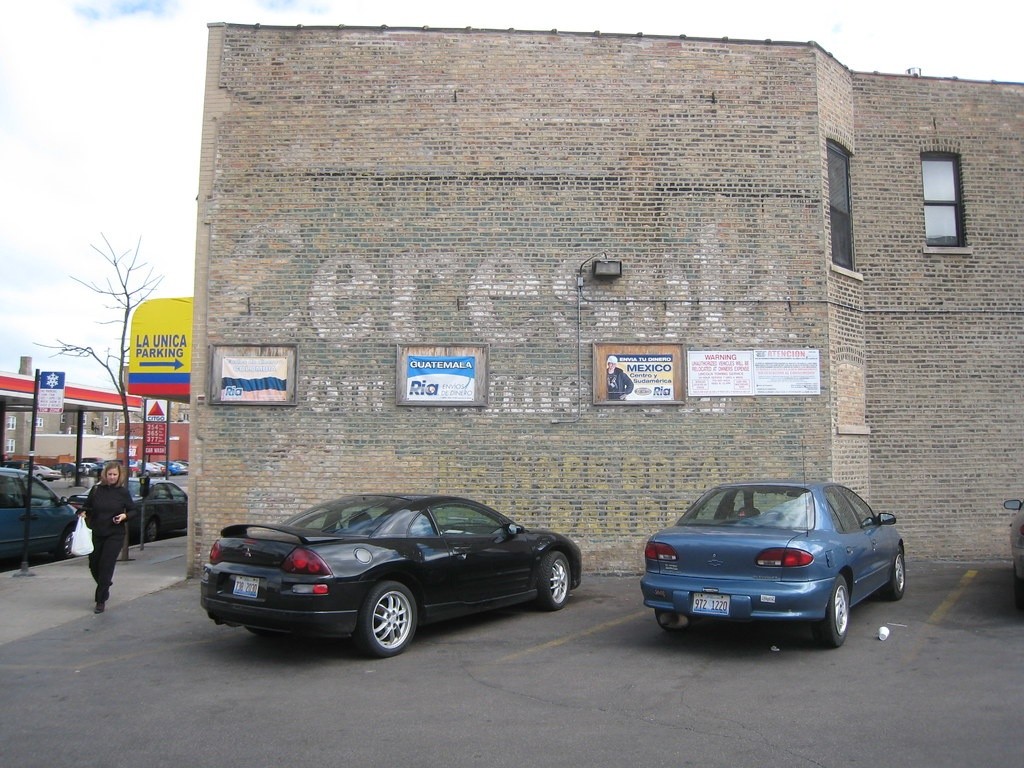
[591,341,688,406]
[205,342,299,407]
[396,344,490,409]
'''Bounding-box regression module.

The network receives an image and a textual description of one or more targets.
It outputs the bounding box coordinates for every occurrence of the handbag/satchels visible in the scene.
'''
[71,516,94,556]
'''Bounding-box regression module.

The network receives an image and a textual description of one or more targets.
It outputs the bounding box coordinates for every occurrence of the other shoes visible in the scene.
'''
[104,587,109,601]
[94,602,105,613]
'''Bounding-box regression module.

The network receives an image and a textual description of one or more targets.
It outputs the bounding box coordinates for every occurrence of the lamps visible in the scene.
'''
[591,258,622,277]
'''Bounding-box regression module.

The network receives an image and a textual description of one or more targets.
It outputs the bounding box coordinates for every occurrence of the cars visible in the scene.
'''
[18,465,62,483]
[66,476,189,545]
[1004,500,1024,607]
[51,462,89,478]
[0,467,81,561]
[3,459,38,469]
[75,460,188,478]
[199,492,583,662]
[640,480,905,651]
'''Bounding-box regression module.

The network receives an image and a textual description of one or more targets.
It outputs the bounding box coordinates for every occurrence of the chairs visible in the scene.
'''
[349,512,372,539]
[738,508,761,523]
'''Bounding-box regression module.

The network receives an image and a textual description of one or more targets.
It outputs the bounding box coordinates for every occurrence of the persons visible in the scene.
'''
[78,463,137,614]
[606,356,633,400]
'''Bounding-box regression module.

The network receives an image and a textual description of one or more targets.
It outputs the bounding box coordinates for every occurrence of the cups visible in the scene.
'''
[879,627,889,641]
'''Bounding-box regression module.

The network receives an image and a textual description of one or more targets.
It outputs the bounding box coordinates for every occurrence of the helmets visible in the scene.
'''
[608,356,617,363]
